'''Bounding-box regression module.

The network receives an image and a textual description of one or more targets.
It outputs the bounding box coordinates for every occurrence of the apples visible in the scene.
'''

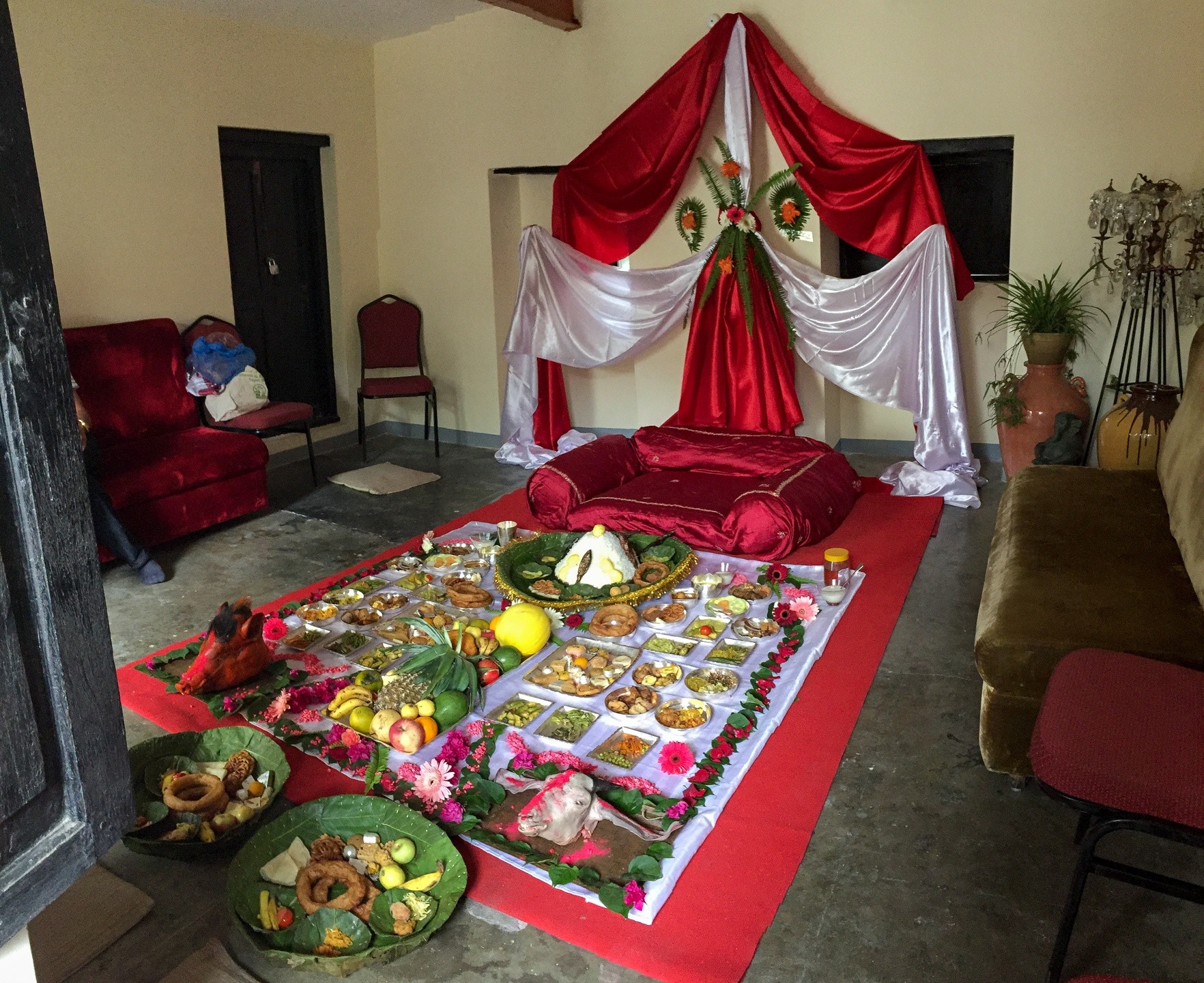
[389,718,424,753]
[211,813,237,834]
[390,837,416,864]
[235,806,254,824]
[379,865,406,890]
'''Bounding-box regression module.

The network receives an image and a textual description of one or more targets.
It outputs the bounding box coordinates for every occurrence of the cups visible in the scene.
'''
[497,521,518,546]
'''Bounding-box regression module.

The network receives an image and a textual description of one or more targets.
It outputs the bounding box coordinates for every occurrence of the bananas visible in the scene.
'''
[328,686,378,719]
[476,637,499,656]
[400,859,445,893]
[199,821,215,842]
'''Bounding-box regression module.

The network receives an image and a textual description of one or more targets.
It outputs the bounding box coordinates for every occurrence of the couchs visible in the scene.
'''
[527,425,861,562]
[973,325,1203,803]
[62,317,267,565]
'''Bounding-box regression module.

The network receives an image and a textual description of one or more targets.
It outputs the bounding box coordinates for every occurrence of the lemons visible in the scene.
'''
[495,603,551,658]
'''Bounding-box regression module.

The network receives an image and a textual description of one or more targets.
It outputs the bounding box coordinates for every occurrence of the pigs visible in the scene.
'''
[174,597,270,696]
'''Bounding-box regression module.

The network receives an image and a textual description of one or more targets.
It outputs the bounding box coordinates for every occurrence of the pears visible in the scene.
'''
[401,698,436,719]
[369,709,401,744]
[349,706,375,734]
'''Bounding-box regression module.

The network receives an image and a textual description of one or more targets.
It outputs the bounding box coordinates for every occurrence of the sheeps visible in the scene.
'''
[495,764,685,846]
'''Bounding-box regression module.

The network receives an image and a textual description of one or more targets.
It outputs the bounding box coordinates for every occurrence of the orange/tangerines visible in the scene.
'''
[413,716,438,744]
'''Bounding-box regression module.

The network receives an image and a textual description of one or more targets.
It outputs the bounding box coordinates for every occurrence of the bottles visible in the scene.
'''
[824,546,850,588]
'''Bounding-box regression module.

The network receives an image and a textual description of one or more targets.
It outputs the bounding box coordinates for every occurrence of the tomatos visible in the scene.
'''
[478,659,500,685]
[700,625,712,635]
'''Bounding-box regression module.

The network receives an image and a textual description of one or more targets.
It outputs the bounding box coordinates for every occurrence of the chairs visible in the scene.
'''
[180,314,319,489]
[356,295,442,461]
[1029,648,1203,981]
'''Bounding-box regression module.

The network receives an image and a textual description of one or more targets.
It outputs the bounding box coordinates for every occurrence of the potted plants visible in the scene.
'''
[984,259,1117,363]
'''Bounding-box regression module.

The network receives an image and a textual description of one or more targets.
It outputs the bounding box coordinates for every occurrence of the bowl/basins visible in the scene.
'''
[470,533,497,550]
[817,579,850,605]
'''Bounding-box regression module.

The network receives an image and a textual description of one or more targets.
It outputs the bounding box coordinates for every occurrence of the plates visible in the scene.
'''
[272,542,779,771]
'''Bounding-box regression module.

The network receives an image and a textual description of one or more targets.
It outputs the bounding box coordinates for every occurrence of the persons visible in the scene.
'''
[67,363,168,586]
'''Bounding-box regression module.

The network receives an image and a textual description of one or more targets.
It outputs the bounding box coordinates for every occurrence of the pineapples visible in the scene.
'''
[375,616,485,716]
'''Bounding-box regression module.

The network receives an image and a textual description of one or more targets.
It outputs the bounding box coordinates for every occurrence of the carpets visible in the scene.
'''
[104,478,949,983]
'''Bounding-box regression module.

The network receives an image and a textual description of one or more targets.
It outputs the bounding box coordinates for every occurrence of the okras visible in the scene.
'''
[360,647,403,669]
